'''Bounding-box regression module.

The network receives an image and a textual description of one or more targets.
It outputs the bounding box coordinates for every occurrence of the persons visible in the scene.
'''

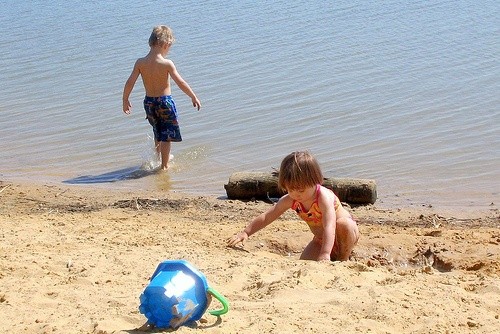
[123,25,200,170]
[226,152,359,262]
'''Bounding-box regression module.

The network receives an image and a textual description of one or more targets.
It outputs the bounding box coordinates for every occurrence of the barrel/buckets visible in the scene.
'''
[137,259,229,330]
